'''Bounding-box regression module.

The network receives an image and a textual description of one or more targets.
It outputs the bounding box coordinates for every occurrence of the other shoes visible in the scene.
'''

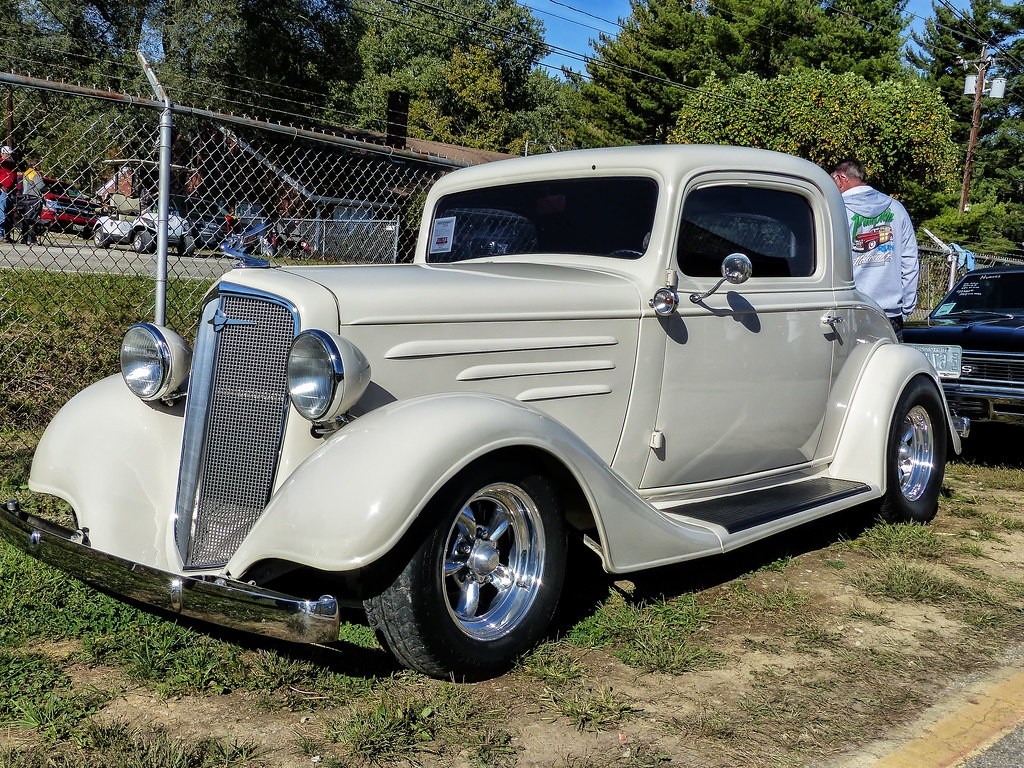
[20,239,26,244]
[3,239,17,243]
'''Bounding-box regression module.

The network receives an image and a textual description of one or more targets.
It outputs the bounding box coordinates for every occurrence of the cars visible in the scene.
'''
[228,197,320,256]
[907,266,1024,436]
[12,172,100,237]
[10,144,972,685]
[146,193,241,251]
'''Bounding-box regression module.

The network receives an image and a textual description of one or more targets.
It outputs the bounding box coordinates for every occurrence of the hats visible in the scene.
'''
[1,146,14,154]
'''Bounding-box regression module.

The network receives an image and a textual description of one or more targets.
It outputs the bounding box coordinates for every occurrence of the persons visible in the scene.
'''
[830,158,918,343]
[0,145,47,246]
